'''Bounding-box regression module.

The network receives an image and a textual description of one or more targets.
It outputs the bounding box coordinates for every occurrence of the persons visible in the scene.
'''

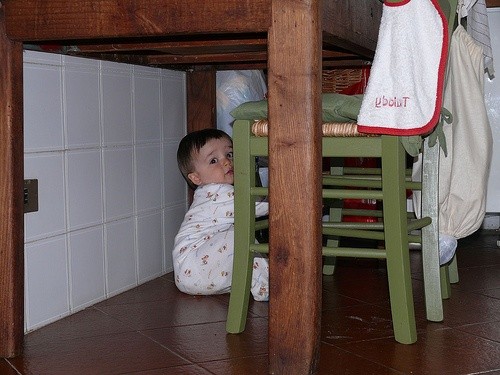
[172,128,269,302]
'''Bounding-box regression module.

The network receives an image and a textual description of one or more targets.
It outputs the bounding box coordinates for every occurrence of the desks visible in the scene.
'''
[0,0,382,375]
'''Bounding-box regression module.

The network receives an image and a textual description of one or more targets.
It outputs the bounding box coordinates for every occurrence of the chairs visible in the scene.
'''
[226,0,495,344]
